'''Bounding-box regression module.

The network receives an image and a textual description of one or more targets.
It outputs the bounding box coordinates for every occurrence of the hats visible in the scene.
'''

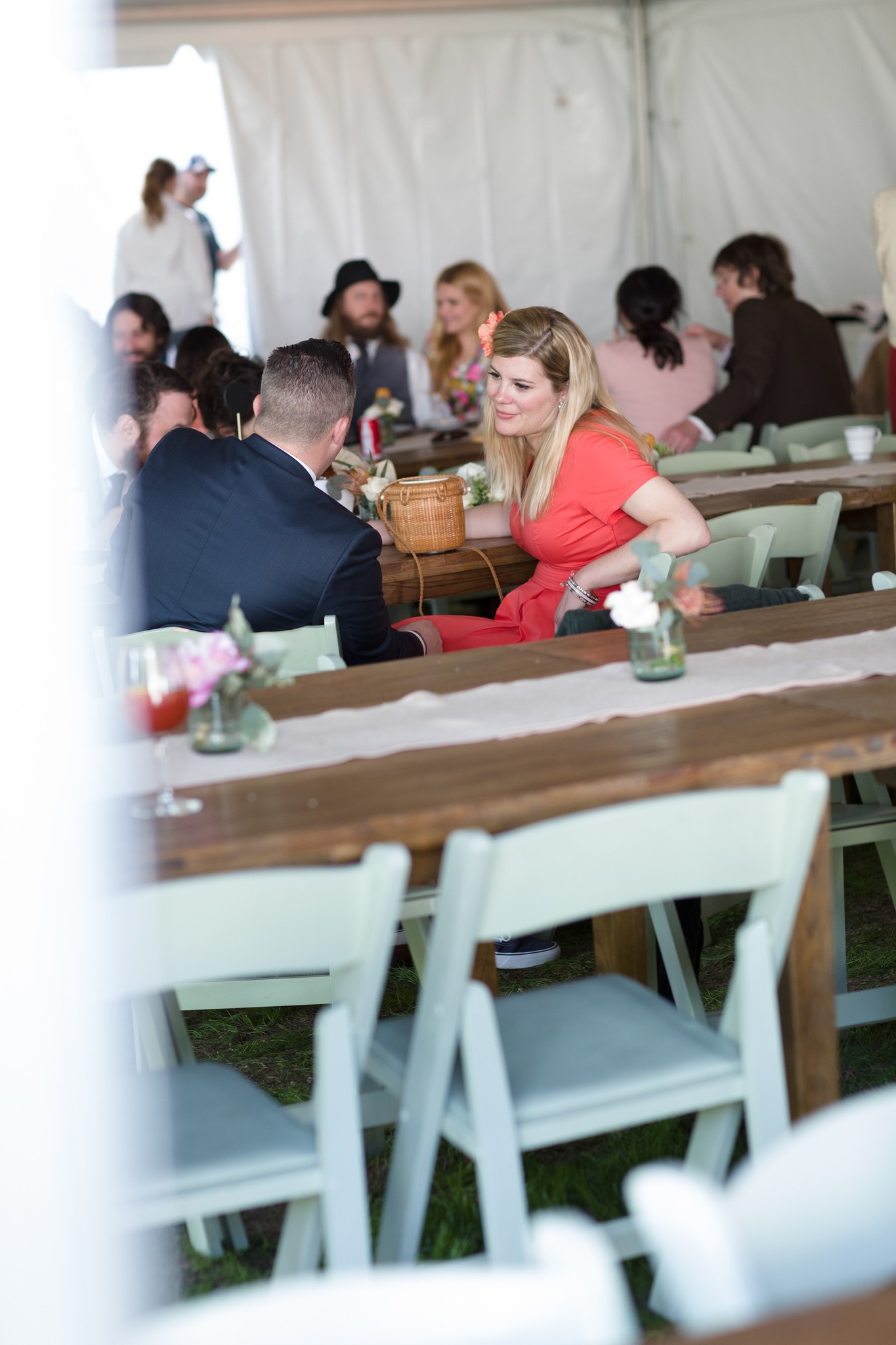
[322,260,400,316]
[175,153,215,174]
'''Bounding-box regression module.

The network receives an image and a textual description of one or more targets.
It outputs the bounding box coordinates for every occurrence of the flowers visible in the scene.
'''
[326,448,389,518]
[443,363,486,423]
[604,579,685,669]
[456,462,491,505]
[375,387,393,438]
[478,310,510,357]
[159,630,254,749]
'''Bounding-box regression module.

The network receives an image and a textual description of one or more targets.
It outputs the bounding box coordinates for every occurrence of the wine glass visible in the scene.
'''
[127,643,203,818]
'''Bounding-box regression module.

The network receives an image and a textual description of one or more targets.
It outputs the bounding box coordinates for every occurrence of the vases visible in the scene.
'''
[378,414,394,446]
[471,484,491,506]
[186,690,245,755]
[358,501,390,522]
[626,614,684,681]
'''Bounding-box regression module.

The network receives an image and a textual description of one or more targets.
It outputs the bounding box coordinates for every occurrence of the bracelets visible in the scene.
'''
[559,569,600,607]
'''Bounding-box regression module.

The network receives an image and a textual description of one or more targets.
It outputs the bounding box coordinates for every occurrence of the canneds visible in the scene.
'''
[357,415,382,461]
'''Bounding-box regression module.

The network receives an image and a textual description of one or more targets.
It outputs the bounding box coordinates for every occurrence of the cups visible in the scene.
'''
[844,425,882,461]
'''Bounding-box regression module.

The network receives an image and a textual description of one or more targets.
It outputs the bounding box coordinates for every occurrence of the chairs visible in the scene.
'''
[123,760,896,1345]
[638,416,896,609]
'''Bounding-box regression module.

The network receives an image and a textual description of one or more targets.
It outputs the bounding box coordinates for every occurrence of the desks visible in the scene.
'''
[123,429,896,1127]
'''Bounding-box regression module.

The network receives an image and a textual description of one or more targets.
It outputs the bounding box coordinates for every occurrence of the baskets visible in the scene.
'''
[377,472,468,555]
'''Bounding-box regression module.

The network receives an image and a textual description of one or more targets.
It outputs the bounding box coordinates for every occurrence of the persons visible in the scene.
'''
[113,158,215,368]
[592,264,720,442]
[418,261,510,427]
[317,259,433,447]
[105,291,397,486]
[101,338,444,677]
[657,232,856,454]
[366,305,711,657]
[855,337,896,434]
[175,154,238,291]
[87,358,192,539]
